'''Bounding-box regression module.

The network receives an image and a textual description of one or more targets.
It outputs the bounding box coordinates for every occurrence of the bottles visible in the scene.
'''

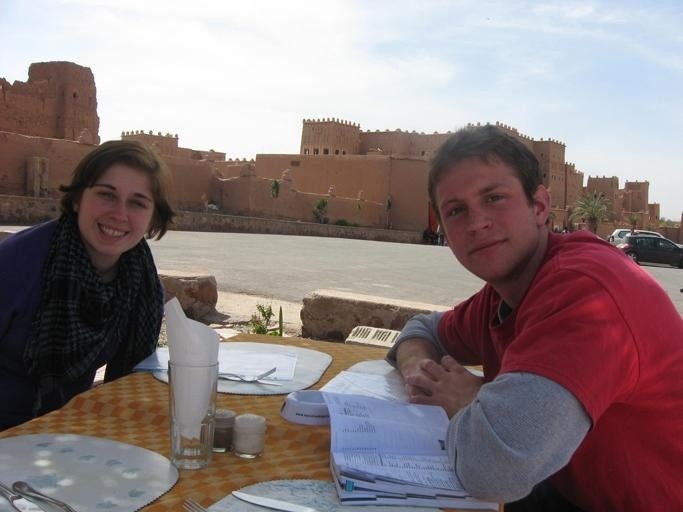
[213,409,267,460]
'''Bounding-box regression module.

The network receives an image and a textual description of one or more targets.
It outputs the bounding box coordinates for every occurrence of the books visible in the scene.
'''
[320,389,503,512]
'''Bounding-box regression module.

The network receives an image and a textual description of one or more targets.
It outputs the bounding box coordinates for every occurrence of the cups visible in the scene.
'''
[168,361,219,472]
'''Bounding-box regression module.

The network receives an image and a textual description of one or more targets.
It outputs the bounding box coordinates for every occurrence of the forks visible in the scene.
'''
[218,367,277,383]
[181,496,207,510]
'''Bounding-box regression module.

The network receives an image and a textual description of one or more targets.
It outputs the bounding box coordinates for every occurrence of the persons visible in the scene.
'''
[553,227,560,233]
[0,142,176,431]
[423,226,437,245]
[437,223,445,246]
[385,126,683,511]
[561,227,569,235]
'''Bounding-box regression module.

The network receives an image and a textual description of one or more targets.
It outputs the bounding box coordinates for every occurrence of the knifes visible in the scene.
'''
[228,488,312,512]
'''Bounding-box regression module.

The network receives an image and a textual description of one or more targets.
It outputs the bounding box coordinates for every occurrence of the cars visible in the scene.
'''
[610,228,683,269]
